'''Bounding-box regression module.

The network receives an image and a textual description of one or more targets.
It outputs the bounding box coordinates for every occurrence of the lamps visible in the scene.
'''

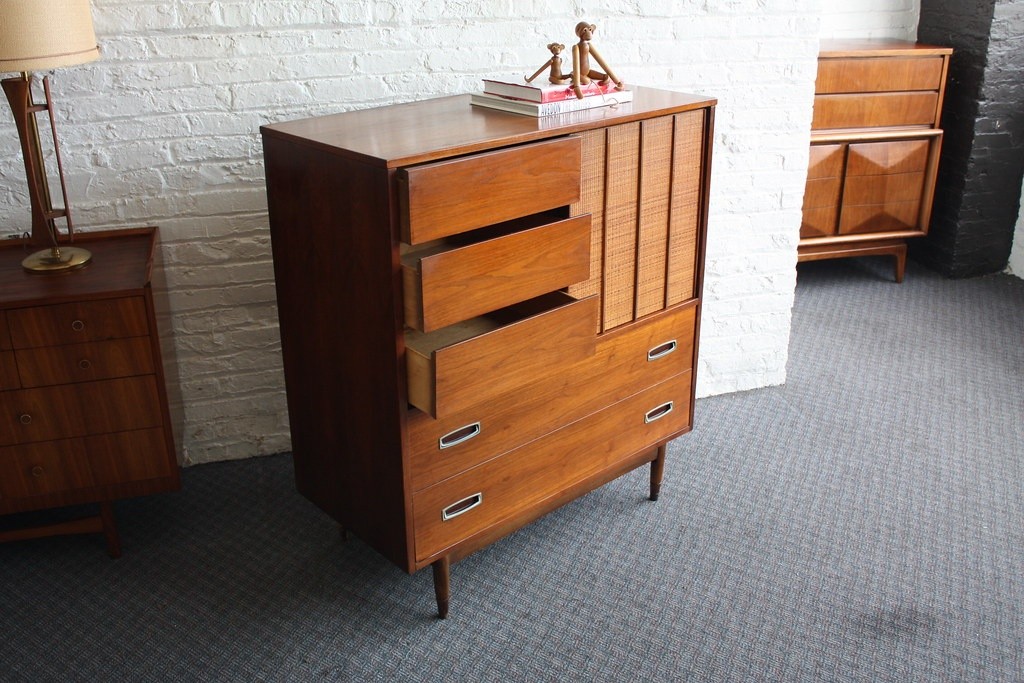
[0,0,101,276]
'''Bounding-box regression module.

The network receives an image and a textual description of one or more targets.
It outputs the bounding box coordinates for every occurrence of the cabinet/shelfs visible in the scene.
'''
[0,226,184,559]
[260,84,718,618]
[795,36,953,283]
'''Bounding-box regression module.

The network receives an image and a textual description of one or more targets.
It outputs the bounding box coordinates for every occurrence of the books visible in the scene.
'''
[468,78,633,118]
